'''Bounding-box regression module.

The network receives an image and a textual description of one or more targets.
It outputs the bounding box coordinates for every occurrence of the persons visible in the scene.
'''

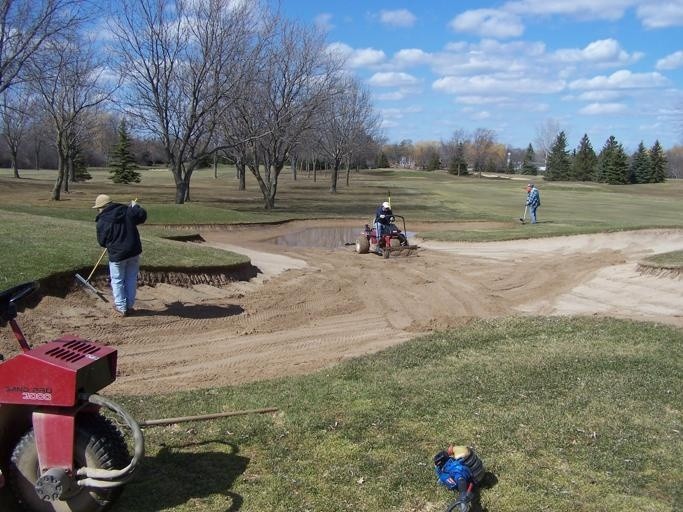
[91,193,146,318]
[373,201,407,251]
[523,182,541,225]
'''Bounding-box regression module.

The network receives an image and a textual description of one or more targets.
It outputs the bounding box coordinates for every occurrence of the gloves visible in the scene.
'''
[131,200,138,207]
[380,215,385,218]
[391,217,394,221]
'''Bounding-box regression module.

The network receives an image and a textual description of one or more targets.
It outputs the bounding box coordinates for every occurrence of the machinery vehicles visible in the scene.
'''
[0,280,144,512]
[356,215,418,259]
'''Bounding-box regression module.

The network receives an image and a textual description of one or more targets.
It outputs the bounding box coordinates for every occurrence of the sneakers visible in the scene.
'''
[112,305,133,315]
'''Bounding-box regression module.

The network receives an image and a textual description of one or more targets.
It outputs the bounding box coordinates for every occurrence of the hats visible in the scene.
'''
[383,202,389,209]
[528,184,534,187]
[93,194,111,209]
